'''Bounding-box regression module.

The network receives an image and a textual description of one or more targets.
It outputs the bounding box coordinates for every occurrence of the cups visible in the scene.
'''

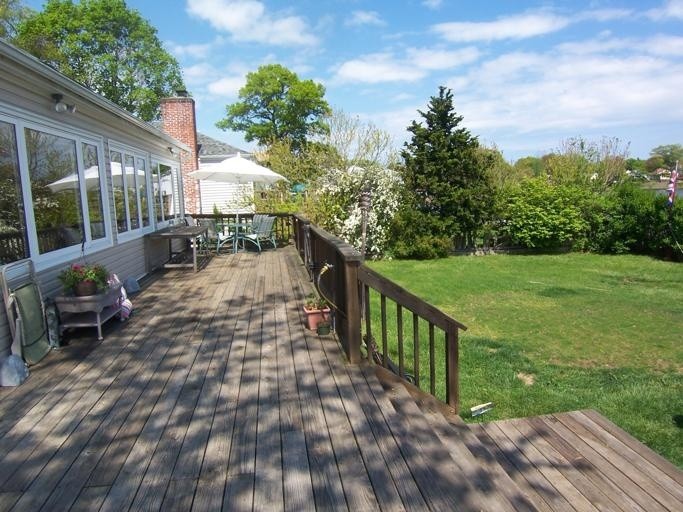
[218,232,223,238]
[229,231,233,235]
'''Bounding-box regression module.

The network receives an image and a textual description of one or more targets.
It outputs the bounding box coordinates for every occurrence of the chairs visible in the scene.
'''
[197,219,218,253]
[0,259,52,365]
[212,214,277,255]
[183,216,212,256]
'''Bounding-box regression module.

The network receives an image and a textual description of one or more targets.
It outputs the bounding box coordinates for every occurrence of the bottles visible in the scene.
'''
[168,214,174,232]
[224,225,229,237]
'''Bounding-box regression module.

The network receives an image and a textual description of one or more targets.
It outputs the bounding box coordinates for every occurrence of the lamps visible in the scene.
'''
[51,93,76,113]
[168,146,178,158]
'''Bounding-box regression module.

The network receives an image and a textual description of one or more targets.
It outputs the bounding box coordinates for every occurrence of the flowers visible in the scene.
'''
[58,263,108,294]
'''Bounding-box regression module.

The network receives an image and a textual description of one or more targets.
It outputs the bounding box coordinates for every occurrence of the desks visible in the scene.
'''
[54,284,125,341]
[144,226,208,273]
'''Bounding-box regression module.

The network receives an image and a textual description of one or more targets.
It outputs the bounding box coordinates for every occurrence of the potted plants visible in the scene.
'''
[304,292,331,330]
[317,299,329,335]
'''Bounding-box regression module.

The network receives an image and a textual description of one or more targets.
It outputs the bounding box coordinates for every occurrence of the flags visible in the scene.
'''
[666,168,679,208]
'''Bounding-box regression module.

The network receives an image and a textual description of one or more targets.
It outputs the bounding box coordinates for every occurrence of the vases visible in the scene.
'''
[73,280,97,296]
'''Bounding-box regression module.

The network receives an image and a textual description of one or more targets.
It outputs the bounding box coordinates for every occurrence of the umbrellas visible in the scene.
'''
[44,161,158,196]
[187,152,290,249]
